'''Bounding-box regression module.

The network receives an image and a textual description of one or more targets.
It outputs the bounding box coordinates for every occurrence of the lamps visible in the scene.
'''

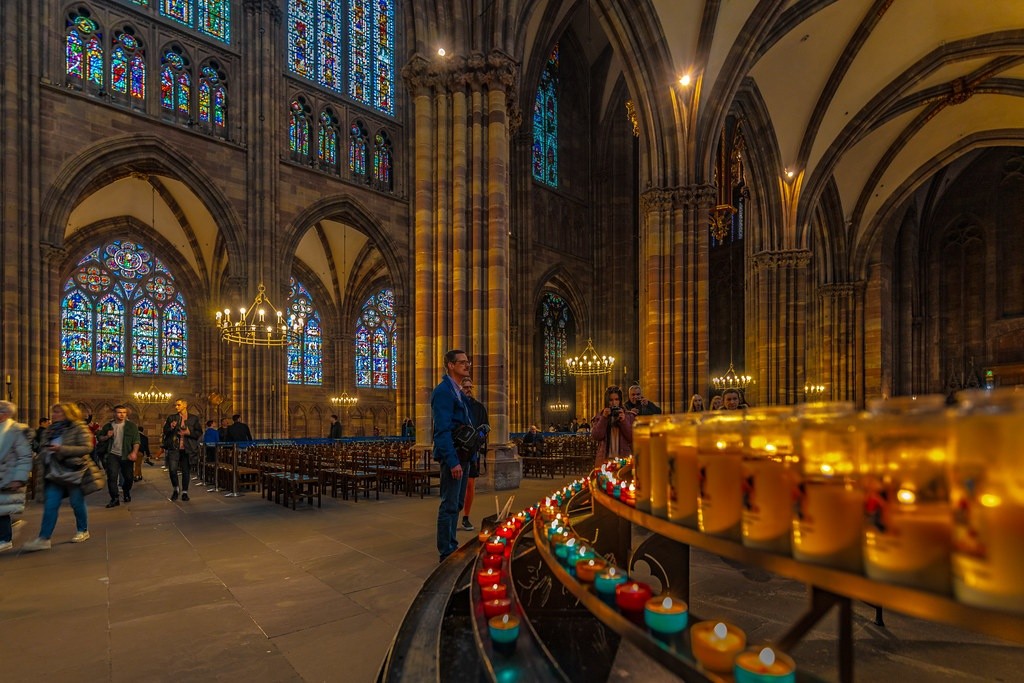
[132,384,172,404]
[214,283,304,349]
[712,366,751,390]
[804,379,824,398]
[328,391,359,409]
[566,340,616,377]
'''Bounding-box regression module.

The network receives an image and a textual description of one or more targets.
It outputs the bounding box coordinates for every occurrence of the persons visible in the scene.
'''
[162,397,203,501]
[31,417,50,452]
[688,394,705,412]
[23,403,105,549]
[623,385,661,415]
[401,418,414,436]
[709,389,749,410]
[591,386,636,468]
[204,414,253,443]
[571,418,591,434]
[461,377,488,530]
[327,414,342,439]
[523,426,544,457]
[430,350,473,563]
[96,405,141,508]
[0,399,34,552]
[132,426,150,481]
[548,423,569,432]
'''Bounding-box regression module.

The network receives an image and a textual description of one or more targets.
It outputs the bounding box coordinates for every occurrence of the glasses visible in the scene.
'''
[606,387,620,392]
[450,360,470,365]
[463,386,474,389]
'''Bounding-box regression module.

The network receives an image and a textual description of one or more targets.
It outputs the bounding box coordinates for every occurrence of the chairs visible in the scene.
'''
[183,432,599,512]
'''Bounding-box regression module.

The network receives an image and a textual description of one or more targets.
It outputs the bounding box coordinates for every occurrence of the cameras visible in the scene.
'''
[610,406,621,417]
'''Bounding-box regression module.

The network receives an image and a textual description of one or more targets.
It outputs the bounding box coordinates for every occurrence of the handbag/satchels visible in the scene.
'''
[44,453,88,484]
[96,422,112,456]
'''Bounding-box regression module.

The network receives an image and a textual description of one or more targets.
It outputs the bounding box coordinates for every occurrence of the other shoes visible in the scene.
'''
[182,493,189,501]
[0,540,13,552]
[461,516,474,531]
[105,497,120,508]
[170,490,178,501]
[123,490,131,502]
[20,537,51,551]
[71,531,90,543]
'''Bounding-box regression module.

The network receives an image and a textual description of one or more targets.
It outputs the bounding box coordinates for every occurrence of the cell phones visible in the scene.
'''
[636,400,642,413]
[41,444,53,447]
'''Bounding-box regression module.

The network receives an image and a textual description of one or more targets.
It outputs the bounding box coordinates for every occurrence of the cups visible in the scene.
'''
[632,388,1024,612]
